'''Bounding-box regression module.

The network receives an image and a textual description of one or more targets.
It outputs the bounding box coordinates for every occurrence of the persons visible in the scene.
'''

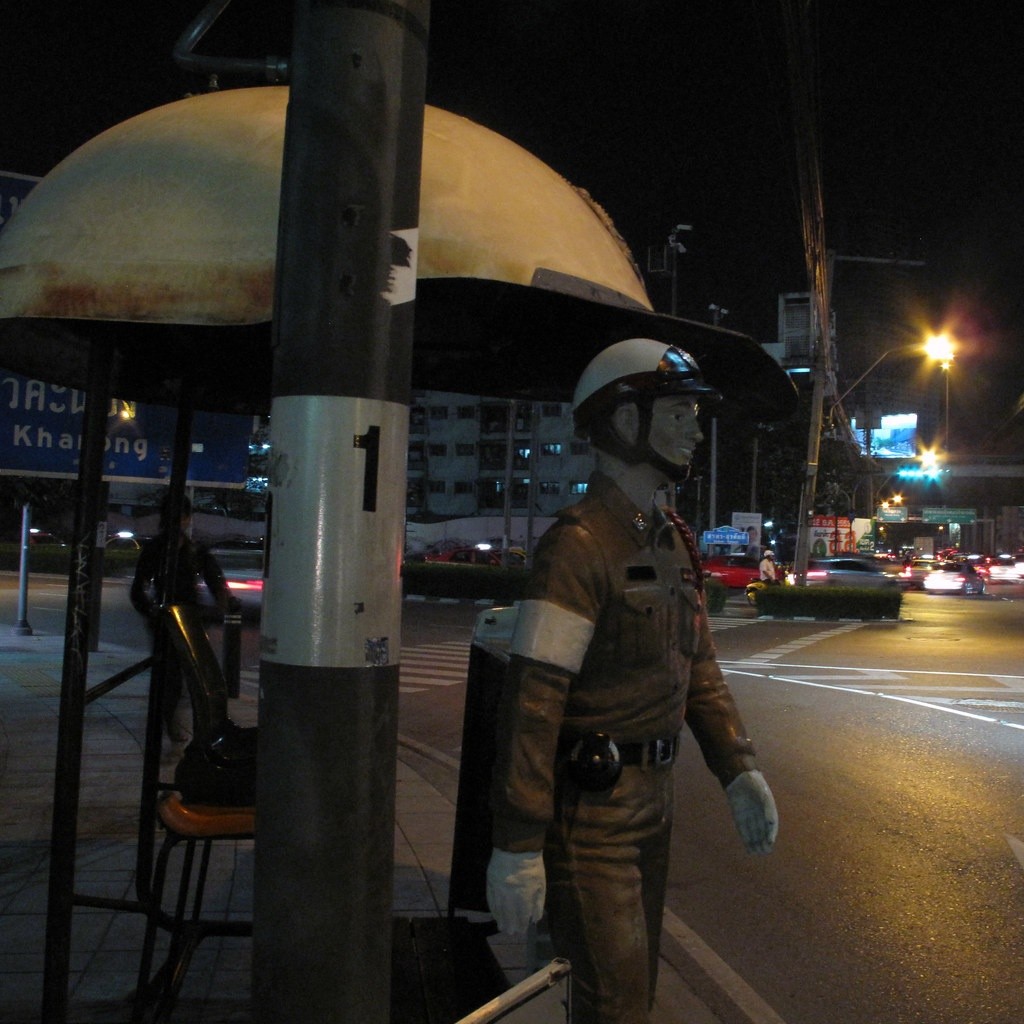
[760,550,781,586]
[485,337,779,1023]
[130,493,237,743]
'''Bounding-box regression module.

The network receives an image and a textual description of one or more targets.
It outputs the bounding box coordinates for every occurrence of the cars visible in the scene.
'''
[425,547,525,570]
[887,549,1024,595]
[793,556,899,591]
[700,555,784,587]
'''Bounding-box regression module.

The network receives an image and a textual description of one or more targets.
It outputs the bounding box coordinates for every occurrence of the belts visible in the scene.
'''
[620,737,678,767]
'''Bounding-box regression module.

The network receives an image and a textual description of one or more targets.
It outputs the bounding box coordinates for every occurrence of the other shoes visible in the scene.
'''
[160,727,187,756]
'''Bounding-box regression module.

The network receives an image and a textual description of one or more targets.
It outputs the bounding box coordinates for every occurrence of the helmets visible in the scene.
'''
[573,338,715,426]
[764,549,774,558]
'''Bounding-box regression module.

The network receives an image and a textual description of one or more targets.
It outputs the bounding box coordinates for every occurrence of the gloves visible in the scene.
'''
[726,770,779,855]
[486,846,547,937]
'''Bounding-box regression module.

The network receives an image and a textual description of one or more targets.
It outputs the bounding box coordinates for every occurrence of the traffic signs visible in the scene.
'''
[922,507,977,525]
[877,507,908,523]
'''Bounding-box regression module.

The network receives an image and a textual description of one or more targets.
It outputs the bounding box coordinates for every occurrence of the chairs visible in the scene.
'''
[137,601,255,1024]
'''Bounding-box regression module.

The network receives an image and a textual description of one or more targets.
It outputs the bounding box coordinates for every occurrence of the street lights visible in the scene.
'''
[792,245,957,571]
